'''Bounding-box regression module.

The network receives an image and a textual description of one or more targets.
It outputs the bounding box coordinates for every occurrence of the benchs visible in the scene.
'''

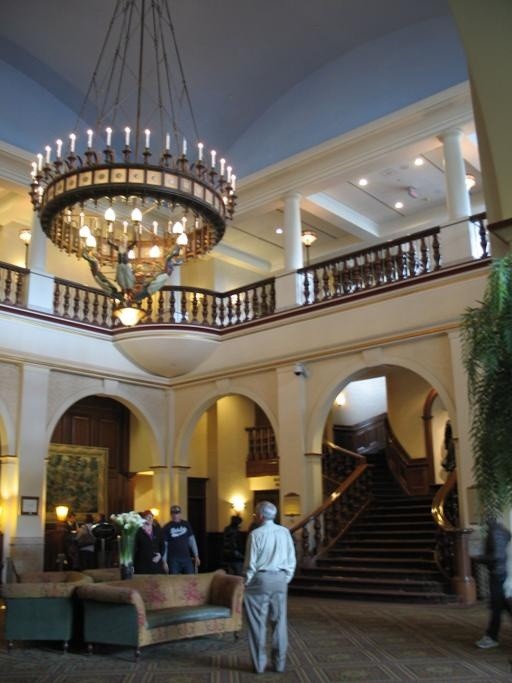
[76,569,246,663]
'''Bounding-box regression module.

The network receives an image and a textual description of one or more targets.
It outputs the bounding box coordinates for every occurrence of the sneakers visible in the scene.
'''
[474,635,500,649]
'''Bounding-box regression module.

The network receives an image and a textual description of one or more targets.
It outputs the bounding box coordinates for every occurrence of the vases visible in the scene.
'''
[120,563,135,580]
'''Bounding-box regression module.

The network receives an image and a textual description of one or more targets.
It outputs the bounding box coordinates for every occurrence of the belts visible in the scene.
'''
[258,569,287,572]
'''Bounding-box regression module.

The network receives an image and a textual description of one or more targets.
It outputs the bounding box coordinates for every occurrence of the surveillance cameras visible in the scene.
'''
[293,362,304,376]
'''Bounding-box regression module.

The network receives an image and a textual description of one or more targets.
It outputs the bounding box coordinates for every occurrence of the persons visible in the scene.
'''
[106,224,138,300]
[138,245,185,299]
[221,515,243,575]
[128,261,155,299]
[242,502,297,674]
[469,518,512,649]
[81,249,124,303]
[63,505,200,574]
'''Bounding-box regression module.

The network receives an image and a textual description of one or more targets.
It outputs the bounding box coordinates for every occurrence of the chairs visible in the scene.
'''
[84,567,135,581]
[1,570,95,655]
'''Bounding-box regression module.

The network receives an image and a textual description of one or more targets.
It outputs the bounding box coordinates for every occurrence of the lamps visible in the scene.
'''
[283,492,301,517]
[27,1,238,332]
[301,230,317,267]
[18,228,31,268]
[54,503,70,570]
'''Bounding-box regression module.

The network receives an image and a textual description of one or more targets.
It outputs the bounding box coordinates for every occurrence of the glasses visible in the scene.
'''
[171,511,181,515]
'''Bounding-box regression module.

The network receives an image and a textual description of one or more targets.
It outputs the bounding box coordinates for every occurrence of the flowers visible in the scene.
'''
[109,510,147,566]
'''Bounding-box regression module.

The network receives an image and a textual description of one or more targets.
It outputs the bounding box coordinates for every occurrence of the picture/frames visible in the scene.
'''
[21,496,39,515]
[46,443,109,524]
